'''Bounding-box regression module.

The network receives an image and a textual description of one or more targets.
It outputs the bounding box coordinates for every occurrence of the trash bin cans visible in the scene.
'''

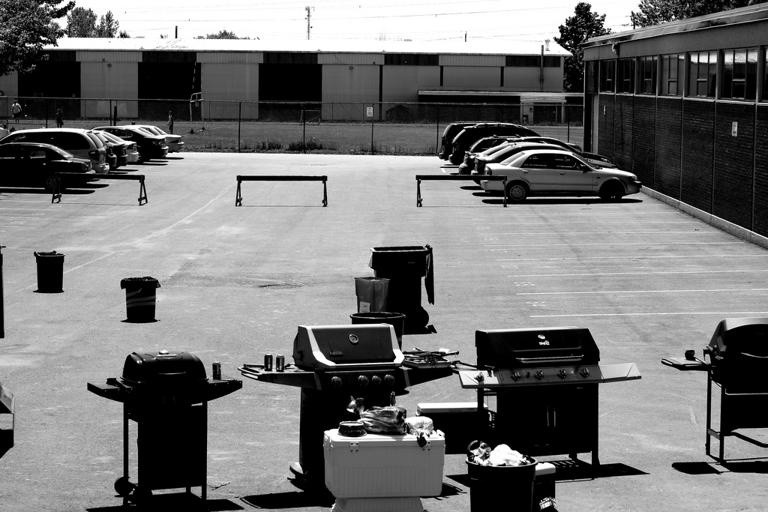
[368,244,434,312]
[465,455,538,512]
[349,311,406,351]
[122,277,158,321]
[36,252,64,290]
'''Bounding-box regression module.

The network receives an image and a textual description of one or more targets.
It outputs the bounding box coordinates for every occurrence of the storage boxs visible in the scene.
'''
[323,428,446,499]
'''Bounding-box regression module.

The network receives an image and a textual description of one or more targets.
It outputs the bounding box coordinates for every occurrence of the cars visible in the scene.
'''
[438,122,642,203]
[0,124,184,193]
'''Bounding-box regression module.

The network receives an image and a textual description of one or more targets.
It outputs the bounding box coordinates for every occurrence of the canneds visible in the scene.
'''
[213,362,221,380]
[264,354,273,371]
[276,354,285,372]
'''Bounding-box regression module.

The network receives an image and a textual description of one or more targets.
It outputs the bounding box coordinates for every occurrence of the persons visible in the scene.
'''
[168,110,175,134]
[55,108,64,127]
[12,100,23,123]
